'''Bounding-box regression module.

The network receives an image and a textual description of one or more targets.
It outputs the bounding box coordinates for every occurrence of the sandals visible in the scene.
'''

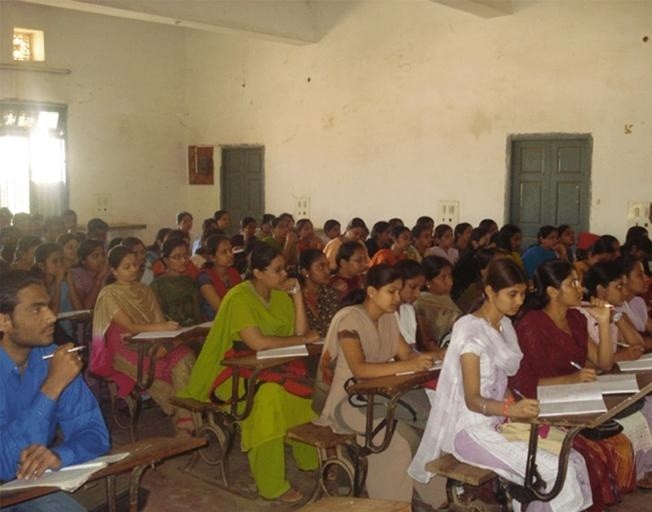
[275,487,304,502]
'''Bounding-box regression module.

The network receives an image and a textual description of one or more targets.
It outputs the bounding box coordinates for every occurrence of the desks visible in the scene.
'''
[294,496,413,512]
[0,436,209,512]
[346,350,446,499]
[510,370,652,511]
[53,308,325,502]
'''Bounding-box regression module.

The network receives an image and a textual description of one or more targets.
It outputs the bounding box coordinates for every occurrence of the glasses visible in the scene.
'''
[168,252,190,260]
[553,278,583,290]
[406,280,425,293]
[348,256,370,263]
[262,265,289,273]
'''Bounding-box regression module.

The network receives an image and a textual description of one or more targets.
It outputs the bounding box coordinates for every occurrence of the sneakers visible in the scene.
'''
[305,464,339,481]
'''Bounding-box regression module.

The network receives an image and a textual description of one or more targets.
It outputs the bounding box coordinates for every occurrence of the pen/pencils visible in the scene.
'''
[616,343,644,350]
[570,362,582,370]
[580,301,614,308]
[513,388,525,398]
[43,346,86,359]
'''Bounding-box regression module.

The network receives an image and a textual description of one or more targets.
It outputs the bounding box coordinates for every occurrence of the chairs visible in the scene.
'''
[0,229,293,511]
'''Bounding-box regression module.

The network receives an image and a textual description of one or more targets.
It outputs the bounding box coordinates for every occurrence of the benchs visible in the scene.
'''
[424,453,510,512]
[284,421,355,503]
